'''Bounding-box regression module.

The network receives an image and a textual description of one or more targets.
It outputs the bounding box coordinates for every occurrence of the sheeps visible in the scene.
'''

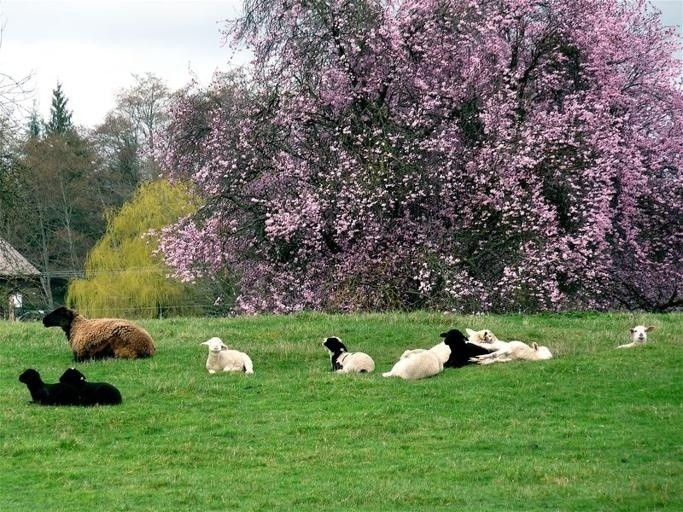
[41,306,157,362]
[380,327,554,381]
[18,367,60,406]
[57,367,123,406]
[614,324,655,349]
[320,335,375,374]
[200,336,254,375]
